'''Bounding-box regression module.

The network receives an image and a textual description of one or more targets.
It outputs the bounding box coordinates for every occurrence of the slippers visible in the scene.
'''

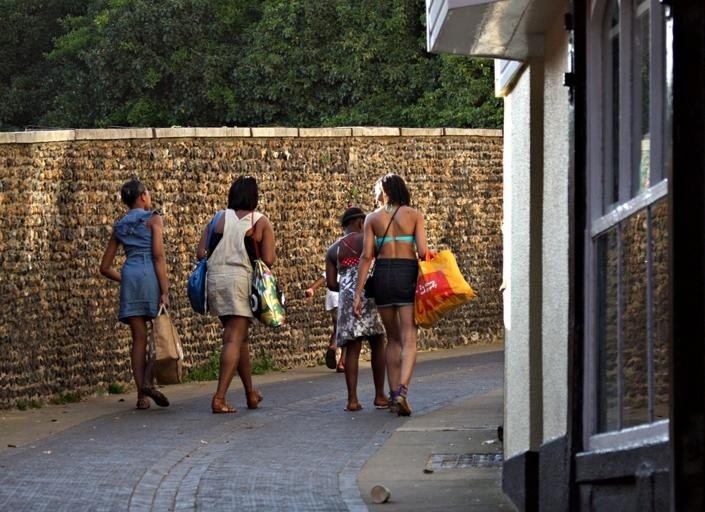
[373,394,392,410]
[342,401,368,412]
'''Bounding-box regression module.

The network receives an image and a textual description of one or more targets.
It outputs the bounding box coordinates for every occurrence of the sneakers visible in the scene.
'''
[137,398,150,410]
[141,383,169,407]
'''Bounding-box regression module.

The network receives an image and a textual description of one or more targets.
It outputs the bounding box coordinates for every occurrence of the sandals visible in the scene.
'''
[336,359,345,373]
[211,396,237,414]
[244,387,264,409]
[388,398,399,414]
[325,345,337,369]
[394,394,411,418]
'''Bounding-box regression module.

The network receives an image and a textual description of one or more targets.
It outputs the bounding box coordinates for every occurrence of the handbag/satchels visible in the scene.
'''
[363,274,375,298]
[150,304,184,363]
[413,248,475,329]
[186,254,208,316]
[249,257,287,329]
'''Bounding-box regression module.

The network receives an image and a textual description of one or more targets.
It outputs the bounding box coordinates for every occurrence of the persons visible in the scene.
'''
[352,173,439,417]
[99,180,172,411]
[327,207,389,410]
[195,175,276,413]
[303,270,346,373]
